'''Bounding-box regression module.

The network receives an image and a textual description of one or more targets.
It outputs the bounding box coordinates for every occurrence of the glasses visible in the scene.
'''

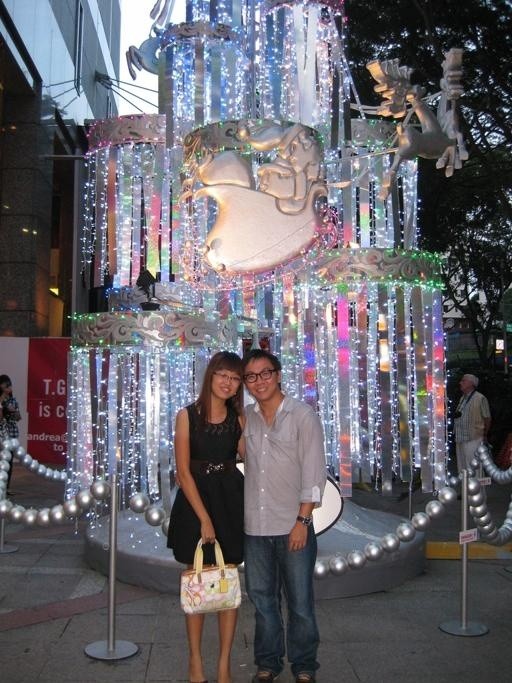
[242,367,278,383]
[213,371,241,383]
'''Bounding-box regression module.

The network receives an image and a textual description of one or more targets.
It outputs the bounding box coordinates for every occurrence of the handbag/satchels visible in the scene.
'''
[178,537,243,616]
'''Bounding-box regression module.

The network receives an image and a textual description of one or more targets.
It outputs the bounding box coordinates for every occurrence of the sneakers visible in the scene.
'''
[251,668,280,683]
[294,668,315,683]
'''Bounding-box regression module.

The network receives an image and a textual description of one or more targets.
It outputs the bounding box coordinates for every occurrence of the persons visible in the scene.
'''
[1,373,22,496]
[164,349,249,683]
[453,371,490,502]
[238,349,330,681]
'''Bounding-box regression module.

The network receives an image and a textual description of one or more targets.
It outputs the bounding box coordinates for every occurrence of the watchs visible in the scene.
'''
[296,514,312,526]
